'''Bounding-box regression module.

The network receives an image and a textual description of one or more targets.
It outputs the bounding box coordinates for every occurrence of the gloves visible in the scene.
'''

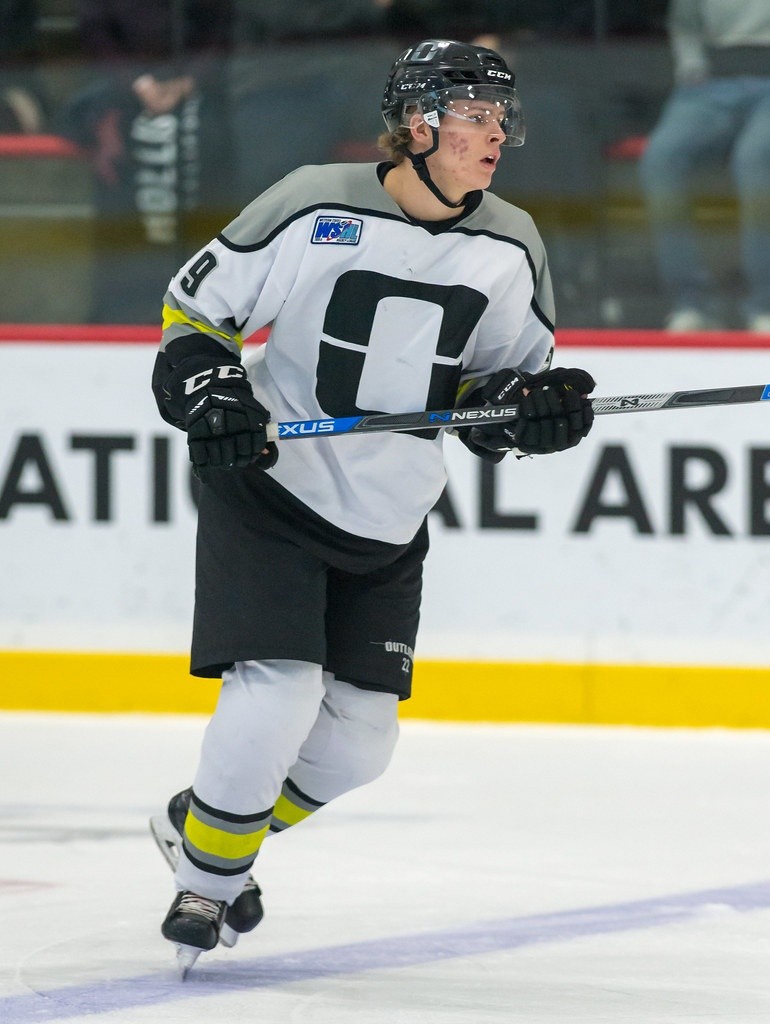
[453,368,595,465]
[151,332,279,483]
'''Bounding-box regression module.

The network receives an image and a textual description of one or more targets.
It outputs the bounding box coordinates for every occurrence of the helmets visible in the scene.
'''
[382,39,526,148]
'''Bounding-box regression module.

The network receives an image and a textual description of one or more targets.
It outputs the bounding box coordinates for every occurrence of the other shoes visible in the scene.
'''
[746,312,766,327]
[661,310,705,327]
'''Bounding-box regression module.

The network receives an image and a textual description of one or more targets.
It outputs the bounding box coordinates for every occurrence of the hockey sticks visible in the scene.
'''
[263,385,770,440]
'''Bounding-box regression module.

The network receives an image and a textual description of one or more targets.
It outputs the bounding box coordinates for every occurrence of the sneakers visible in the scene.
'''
[150,786,264,949]
[161,889,227,982]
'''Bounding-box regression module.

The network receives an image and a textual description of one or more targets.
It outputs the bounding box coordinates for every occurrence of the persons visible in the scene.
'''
[630,0,770,335]
[151,38,596,982]
[81,0,624,321]
